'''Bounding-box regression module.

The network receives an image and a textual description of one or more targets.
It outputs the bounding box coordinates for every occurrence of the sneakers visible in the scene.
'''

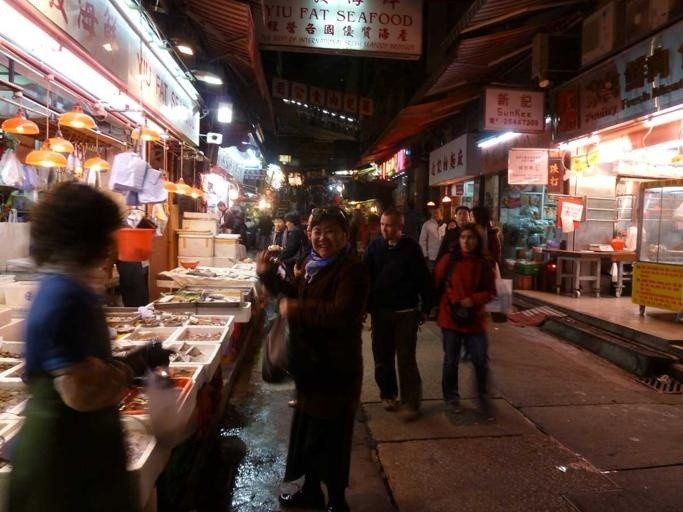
[382,398,396,410]
[401,403,419,423]
[446,400,463,424]
[479,400,495,421]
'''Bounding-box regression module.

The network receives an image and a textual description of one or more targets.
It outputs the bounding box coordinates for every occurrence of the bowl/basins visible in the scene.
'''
[179,260,200,269]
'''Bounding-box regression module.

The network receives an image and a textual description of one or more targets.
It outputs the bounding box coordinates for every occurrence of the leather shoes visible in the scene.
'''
[325,497,349,512]
[279,486,326,511]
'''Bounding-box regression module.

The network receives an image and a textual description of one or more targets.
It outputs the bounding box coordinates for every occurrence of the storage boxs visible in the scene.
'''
[177,257,211,267]
[178,233,214,257]
[0,319,28,511]
[117,365,206,512]
[163,342,218,383]
[518,262,544,274]
[514,273,532,290]
[185,313,234,338]
[181,212,217,233]
[173,326,230,357]
[215,234,240,257]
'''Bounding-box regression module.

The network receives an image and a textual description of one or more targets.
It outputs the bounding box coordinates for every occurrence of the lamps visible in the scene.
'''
[173,40,193,55]
[162,150,178,192]
[426,188,436,206]
[58,103,96,128]
[441,184,451,202]
[176,145,191,194]
[39,122,74,153]
[210,101,232,124]
[185,158,204,199]
[25,82,68,167]
[130,118,161,141]
[83,136,110,170]
[2,99,39,134]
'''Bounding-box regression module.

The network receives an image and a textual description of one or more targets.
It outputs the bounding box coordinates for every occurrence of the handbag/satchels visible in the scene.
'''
[261,261,304,384]
[294,229,311,262]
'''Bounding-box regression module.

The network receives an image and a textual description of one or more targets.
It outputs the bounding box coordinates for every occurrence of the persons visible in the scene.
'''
[446,220,457,231]
[216,202,235,229]
[280,213,311,264]
[266,210,290,252]
[470,206,501,267]
[420,224,499,413]
[419,206,447,274]
[256,207,363,511]
[361,208,432,421]
[11,181,174,511]
[435,207,470,263]
